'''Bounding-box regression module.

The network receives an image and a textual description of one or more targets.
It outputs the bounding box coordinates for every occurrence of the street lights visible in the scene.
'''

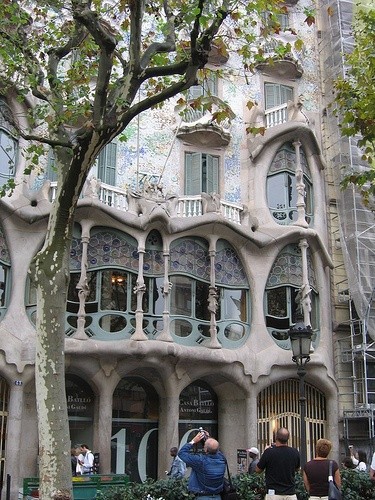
[288,308,313,467]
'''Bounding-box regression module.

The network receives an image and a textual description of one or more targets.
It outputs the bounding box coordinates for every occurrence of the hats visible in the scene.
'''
[246,447,259,455]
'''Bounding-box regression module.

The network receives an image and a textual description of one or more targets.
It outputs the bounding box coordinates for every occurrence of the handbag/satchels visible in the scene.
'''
[224,458,240,500]
[329,460,344,500]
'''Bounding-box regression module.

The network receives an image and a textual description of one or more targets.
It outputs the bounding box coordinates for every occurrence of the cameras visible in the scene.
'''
[348,445,353,450]
[198,427,206,446]
[271,443,276,448]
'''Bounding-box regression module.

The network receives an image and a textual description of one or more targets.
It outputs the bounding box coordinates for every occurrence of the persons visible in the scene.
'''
[246,447,259,475]
[255,427,300,500]
[178,430,227,500]
[342,445,375,480]
[303,439,340,500]
[71,445,96,475]
[165,447,187,479]
